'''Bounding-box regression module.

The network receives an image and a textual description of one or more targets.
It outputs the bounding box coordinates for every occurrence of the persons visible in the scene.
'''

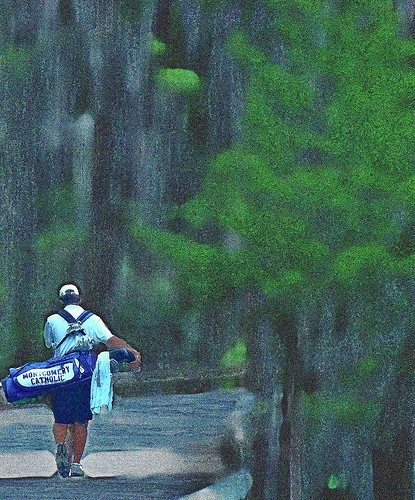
[42,284,142,478]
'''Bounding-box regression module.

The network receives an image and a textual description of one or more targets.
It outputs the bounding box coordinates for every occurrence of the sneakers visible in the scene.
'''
[55,443,70,479]
[70,464,84,476]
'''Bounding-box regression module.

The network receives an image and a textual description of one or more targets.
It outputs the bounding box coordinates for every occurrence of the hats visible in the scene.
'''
[59,284,80,299]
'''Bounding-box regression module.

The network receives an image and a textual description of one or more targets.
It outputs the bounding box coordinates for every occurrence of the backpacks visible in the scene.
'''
[2,310,132,403]
